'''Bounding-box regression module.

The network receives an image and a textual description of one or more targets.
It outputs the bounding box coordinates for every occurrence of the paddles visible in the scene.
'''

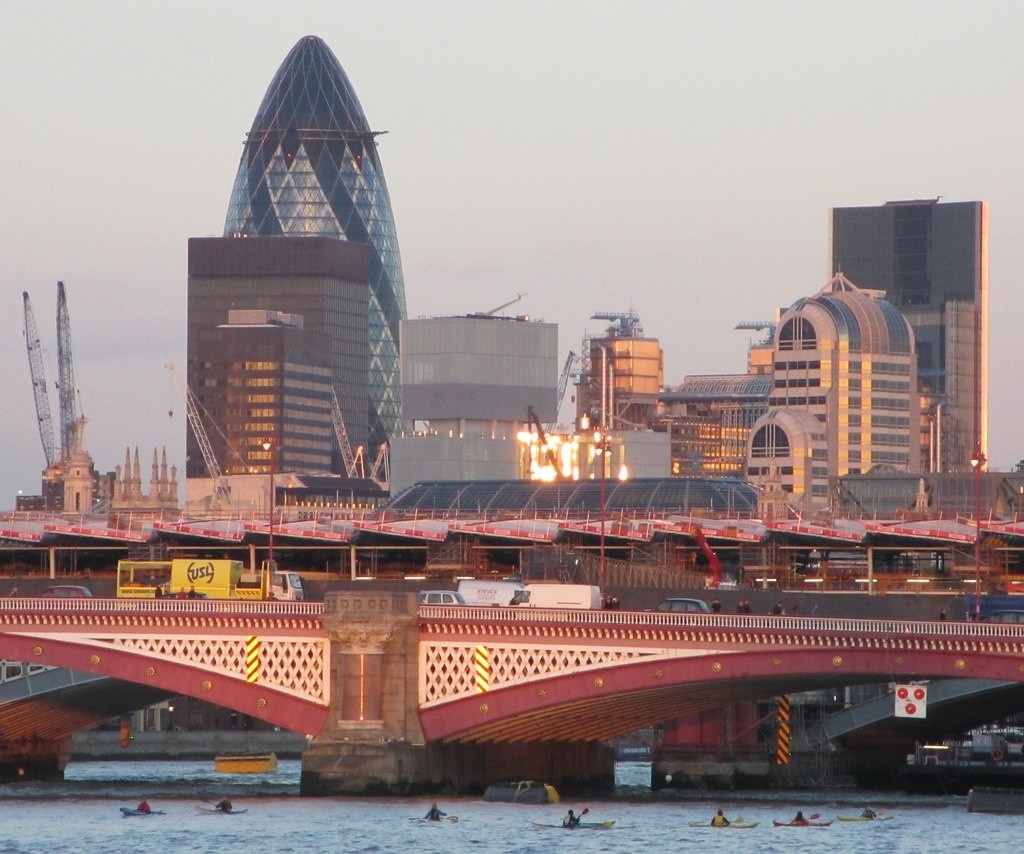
[409,816,458,820]
[579,808,589,817]
[729,819,744,823]
[807,813,819,820]
[876,813,884,816]
[202,797,217,807]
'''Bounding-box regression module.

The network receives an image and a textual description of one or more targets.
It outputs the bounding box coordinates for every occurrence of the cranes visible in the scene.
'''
[162,355,236,507]
[526,405,564,481]
[20,290,56,469]
[57,279,76,458]
[555,349,580,416]
[328,384,361,479]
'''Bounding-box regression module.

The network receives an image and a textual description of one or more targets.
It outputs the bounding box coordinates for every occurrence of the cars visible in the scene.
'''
[417,592,463,605]
[35,585,92,599]
[979,609,1024,627]
[651,596,709,614]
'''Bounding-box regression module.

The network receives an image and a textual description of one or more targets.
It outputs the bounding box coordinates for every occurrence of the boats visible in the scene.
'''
[532,820,617,828]
[120,805,164,815]
[688,821,759,828]
[837,815,892,820]
[195,805,250,814]
[772,820,833,826]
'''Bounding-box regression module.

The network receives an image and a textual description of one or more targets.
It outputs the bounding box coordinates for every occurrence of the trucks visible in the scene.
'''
[115,557,305,602]
[456,577,603,610]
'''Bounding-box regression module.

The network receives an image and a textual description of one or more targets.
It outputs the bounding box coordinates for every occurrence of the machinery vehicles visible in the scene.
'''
[689,527,723,593]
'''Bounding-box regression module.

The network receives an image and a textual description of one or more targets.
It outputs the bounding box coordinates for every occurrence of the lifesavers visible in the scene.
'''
[991,748,1003,761]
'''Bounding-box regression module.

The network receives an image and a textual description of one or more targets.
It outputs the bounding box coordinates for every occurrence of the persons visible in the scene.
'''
[737,599,750,613]
[7,588,18,598]
[604,595,620,609]
[792,811,806,821]
[266,592,279,601]
[772,601,798,615]
[137,799,150,812]
[712,600,721,612]
[215,799,232,812]
[563,809,580,826]
[861,805,877,818]
[710,810,730,825]
[939,607,946,621]
[425,803,447,820]
[810,604,819,615]
[178,585,196,599]
[155,582,163,597]
